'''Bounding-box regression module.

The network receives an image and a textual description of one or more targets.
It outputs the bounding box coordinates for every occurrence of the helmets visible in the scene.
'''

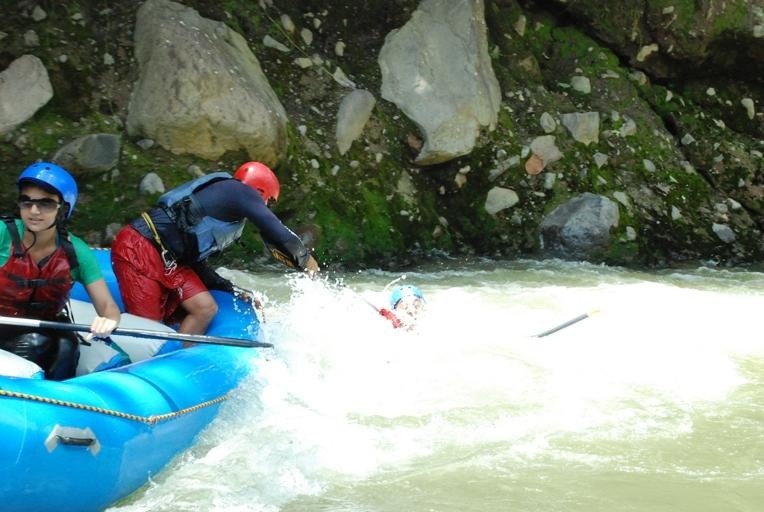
[388,284,426,310]
[232,161,280,206]
[16,161,79,222]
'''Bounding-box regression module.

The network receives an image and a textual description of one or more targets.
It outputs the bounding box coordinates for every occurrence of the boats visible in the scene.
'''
[0,243,273,512]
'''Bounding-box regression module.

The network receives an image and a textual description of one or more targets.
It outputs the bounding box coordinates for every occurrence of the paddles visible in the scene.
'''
[0,316,273,347]
[259,229,400,326]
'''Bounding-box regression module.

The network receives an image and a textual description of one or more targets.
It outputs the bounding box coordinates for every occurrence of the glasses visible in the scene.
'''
[15,199,63,213]
[398,300,423,310]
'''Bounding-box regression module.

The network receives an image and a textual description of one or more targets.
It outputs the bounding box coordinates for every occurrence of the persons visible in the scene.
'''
[108,160,321,349]
[387,284,428,321]
[0,160,121,382]
